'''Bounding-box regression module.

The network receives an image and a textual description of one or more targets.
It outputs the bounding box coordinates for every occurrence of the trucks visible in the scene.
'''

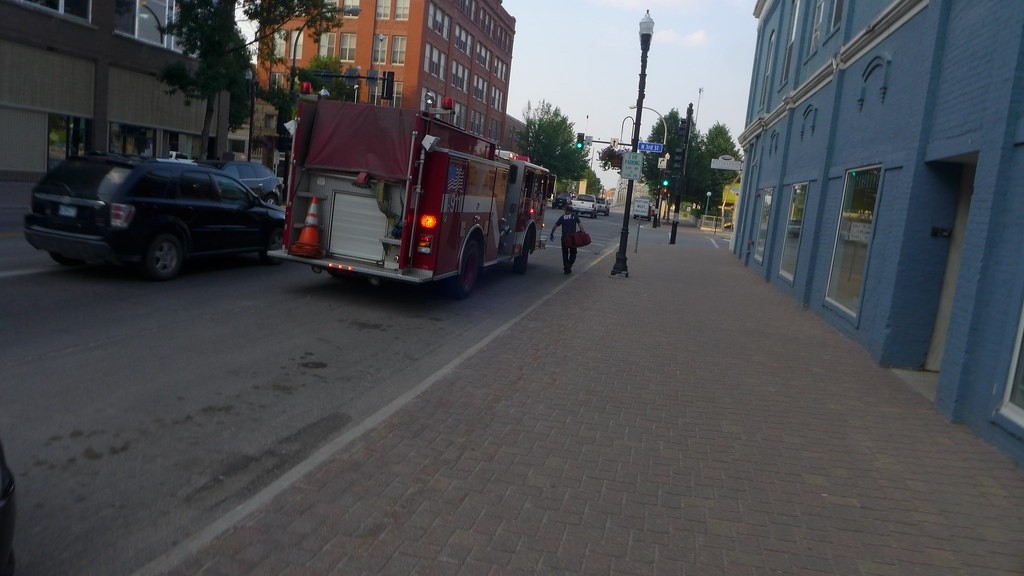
[633,197,654,221]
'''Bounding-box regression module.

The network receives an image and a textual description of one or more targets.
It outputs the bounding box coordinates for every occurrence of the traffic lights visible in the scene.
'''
[677,115,690,145]
[575,132,585,151]
[674,145,685,175]
[662,170,672,191]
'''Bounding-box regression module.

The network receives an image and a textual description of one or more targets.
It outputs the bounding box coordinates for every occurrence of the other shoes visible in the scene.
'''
[563,267,572,274]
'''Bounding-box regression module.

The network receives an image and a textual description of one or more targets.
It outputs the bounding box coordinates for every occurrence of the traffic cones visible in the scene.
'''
[289,195,323,260]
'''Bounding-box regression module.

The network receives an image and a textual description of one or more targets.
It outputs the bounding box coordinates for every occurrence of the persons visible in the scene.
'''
[550,206,585,274]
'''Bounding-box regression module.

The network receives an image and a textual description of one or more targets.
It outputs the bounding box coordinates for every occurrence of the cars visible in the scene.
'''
[552,191,570,210]
[596,198,612,216]
[195,159,282,212]
[20,151,288,280]
[787,218,802,236]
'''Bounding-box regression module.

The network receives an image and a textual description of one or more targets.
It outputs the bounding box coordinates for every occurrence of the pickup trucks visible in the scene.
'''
[571,194,598,217]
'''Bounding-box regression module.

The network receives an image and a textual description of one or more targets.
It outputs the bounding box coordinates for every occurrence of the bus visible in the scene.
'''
[547,173,557,208]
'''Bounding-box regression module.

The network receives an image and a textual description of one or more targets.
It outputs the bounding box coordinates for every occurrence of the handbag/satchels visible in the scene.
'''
[561,231,591,248]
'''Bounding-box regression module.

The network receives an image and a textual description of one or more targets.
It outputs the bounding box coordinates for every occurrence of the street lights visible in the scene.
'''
[628,104,667,227]
[701,191,711,229]
[284,5,361,96]
[609,9,655,277]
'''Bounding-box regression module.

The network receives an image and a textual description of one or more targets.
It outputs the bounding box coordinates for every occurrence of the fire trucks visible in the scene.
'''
[268,99,561,305]
